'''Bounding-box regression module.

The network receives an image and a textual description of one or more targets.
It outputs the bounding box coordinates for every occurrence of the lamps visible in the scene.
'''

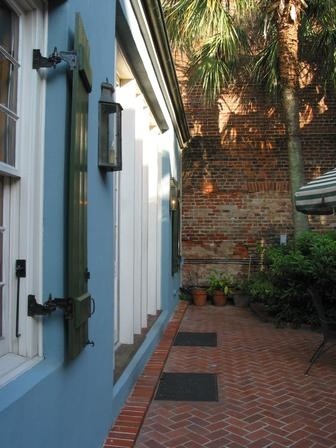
[96,82,123,172]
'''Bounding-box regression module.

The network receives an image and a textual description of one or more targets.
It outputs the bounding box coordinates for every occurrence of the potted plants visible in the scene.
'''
[208,268,230,305]
[228,273,252,307]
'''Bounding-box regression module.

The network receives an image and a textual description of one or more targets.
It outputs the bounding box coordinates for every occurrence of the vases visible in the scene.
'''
[192,288,208,305]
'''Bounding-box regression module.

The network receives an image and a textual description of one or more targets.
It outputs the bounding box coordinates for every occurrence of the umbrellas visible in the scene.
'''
[291,167,336,215]
